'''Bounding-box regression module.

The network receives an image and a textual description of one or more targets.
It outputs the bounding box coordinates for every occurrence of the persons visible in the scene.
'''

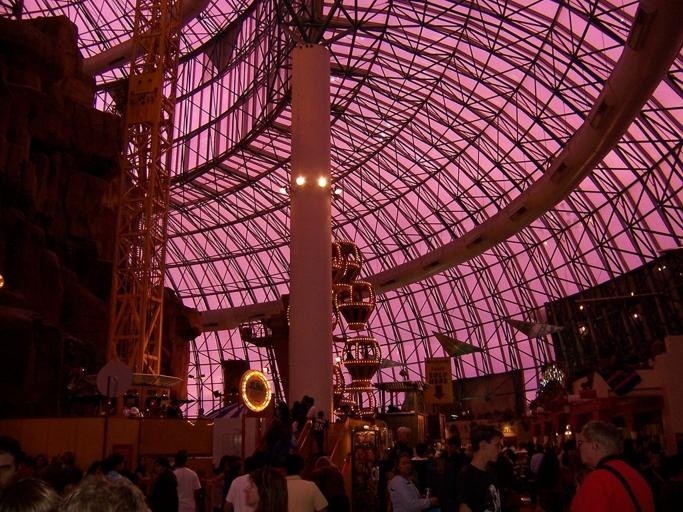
[0,385,681,512]
